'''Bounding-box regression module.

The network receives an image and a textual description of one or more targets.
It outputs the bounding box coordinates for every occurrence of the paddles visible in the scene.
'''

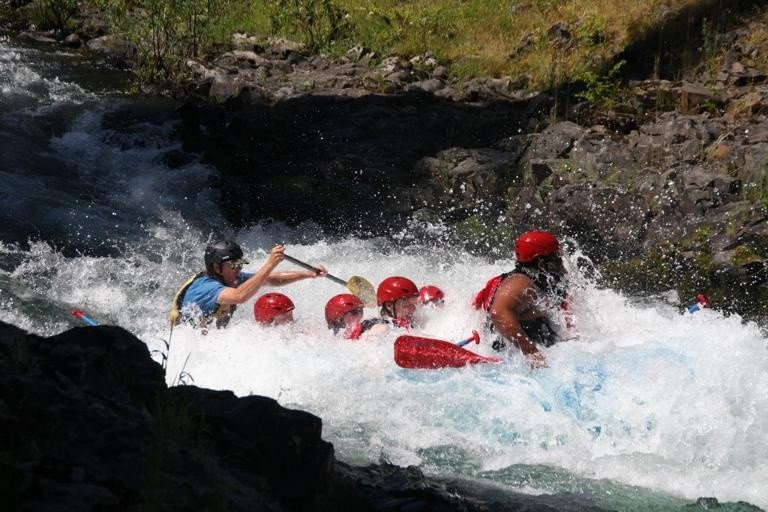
[393,335,497,369]
[283,253,378,309]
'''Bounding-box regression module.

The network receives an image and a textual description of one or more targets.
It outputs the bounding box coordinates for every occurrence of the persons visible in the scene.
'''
[377,275,421,330]
[168,237,330,333]
[418,284,447,310]
[253,292,296,328]
[325,294,390,342]
[470,231,577,374]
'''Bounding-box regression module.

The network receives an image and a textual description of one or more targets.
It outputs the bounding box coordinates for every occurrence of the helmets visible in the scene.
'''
[204,238,250,275]
[324,275,446,330]
[253,292,297,326]
[514,230,560,264]
